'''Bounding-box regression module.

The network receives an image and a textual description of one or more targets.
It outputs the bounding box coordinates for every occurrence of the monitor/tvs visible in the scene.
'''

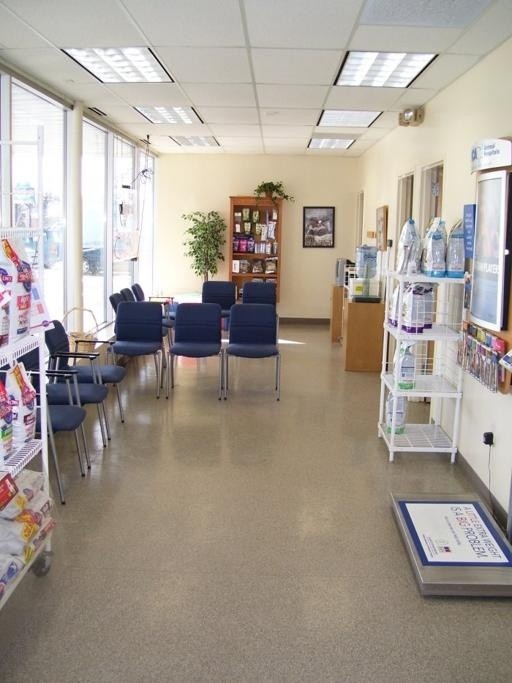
[336,257,355,287]
[466,170,510,332]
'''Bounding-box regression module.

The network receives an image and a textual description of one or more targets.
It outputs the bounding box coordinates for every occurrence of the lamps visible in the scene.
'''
[398,109,424,127]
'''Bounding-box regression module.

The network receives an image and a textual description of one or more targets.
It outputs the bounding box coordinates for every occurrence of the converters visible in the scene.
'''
[483,432,493,444]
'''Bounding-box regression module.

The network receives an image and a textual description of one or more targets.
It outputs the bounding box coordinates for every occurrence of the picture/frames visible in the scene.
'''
[375,207,387,251]
[303,206,335,248]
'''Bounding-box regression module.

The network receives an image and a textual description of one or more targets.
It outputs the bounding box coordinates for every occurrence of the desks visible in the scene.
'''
[330,284,385,372]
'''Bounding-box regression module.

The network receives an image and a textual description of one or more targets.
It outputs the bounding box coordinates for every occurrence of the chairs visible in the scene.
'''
[1,345,85,506]
[224,302,280,402]
[101,301,163,400]
[201,281,237,340]
[120,288,171,356]
[164,302,225,400]
[130,282,176,342]
[45,319,128,441]
[109,292,166,370]
[15,347,109,469]
[243,282,280,345]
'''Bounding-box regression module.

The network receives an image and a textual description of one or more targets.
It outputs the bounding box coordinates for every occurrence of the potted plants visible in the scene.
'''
[254,181,295,208]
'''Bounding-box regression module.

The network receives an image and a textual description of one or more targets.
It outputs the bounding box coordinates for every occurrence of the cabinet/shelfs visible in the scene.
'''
[0,125,54,611]
[227,195,283,303]
[378,239,470,464]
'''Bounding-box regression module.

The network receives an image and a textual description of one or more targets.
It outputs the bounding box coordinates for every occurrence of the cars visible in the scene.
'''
[80,244,156,278]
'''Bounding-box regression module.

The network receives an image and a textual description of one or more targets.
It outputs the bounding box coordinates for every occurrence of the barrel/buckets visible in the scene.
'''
[356,246,377,278]
